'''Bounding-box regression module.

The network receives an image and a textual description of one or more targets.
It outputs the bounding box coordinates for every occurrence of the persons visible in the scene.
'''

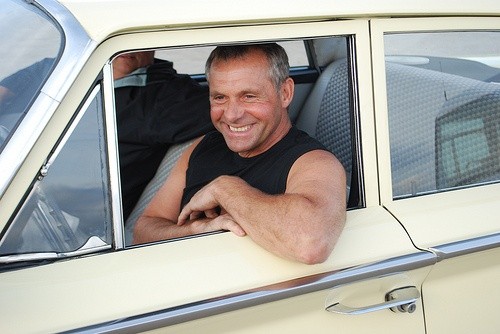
[133,43,347,265]
[0,50,217,230]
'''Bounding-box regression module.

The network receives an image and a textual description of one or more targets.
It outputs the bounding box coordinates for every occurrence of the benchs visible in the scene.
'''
[295,59,500,204]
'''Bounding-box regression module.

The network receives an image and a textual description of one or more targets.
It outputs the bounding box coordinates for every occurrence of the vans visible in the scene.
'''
[1,0,499,334]
